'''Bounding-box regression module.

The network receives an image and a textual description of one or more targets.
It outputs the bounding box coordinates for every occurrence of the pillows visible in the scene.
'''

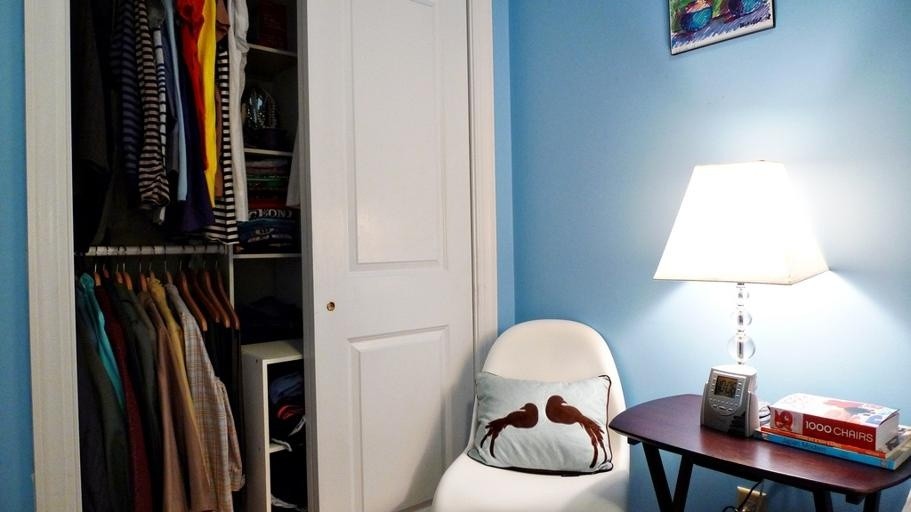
[467,370,614,478]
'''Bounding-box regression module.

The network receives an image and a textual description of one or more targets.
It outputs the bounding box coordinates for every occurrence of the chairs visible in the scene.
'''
[431,319,631,510]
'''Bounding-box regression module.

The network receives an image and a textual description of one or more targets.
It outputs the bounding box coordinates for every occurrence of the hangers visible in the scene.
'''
[74,239,241,332]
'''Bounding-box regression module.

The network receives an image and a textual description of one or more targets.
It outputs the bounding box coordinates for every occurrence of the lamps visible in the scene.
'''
[654,162,830,417]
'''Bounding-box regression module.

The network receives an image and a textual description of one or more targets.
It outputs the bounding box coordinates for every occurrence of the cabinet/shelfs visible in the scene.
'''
[24,0,497,510]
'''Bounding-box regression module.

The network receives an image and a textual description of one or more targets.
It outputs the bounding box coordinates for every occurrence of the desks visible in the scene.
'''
[608,393,911,509]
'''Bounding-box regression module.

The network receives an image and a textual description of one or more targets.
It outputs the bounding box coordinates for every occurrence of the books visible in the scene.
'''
[762,422,911,458]
[755,421,911,471]
[768,391,903,451]
